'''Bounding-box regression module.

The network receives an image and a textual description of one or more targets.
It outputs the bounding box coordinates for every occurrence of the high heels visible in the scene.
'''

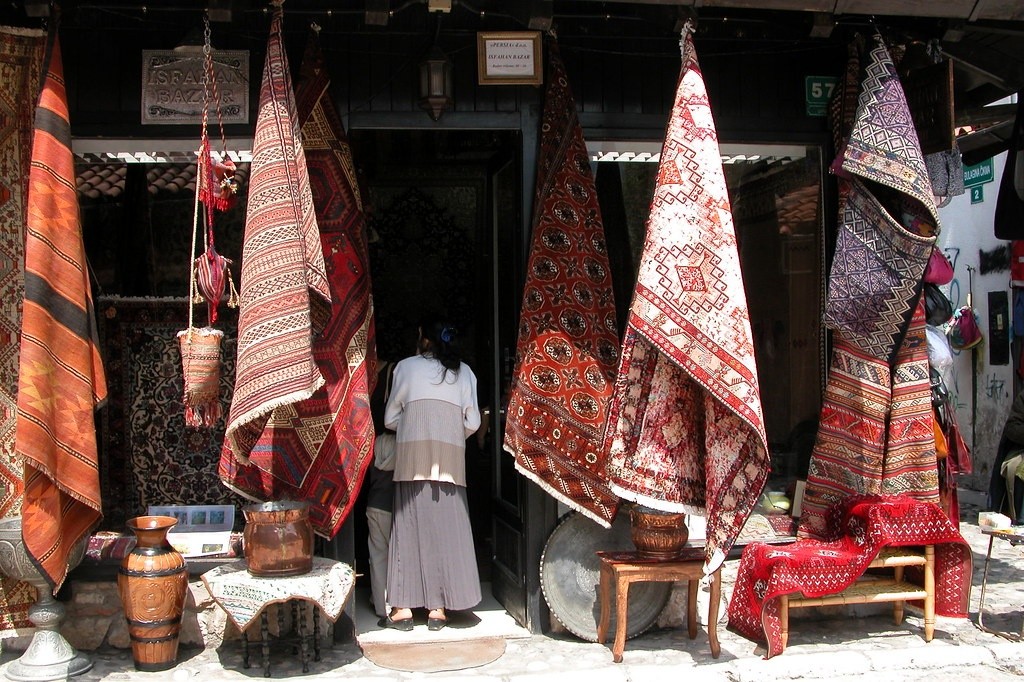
[377,616,414,631]
[428,616,450,630]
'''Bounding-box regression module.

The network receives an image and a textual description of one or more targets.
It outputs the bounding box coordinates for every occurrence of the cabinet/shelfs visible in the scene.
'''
[780,544,935,653]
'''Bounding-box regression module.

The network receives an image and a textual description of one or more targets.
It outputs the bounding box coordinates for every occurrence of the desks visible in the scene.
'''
[200,556,356,678]
[595,547,726,664]
[976,530,1024,641]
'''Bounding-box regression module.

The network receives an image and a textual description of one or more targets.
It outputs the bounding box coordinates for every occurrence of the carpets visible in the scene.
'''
[785,182,974,543]
[724,494,975,660]
[504,29,623,530]
[359,636,507,672]
[224,0,334,468]
[220,33,379,541]
[96,294,255,532]
[0,22,48,638]
[15,9,108,596]
[598,16,771,578]
[819,15,940,363]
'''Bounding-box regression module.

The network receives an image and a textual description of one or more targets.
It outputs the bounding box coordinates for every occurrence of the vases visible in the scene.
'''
[116,517,189,673]
[629,502,689,560]
[240,501,315,578]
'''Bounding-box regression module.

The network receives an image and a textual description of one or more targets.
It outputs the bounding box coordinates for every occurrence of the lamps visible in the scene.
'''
[419,44,453,122]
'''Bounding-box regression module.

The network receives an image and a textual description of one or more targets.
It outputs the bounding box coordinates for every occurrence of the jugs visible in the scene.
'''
[758,491,791,514]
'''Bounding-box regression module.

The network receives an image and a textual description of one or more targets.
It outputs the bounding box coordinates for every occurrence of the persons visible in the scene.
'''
[366,306,490,632]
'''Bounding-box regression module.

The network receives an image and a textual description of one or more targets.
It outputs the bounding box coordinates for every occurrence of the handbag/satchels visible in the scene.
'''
[373,433,397,471]
